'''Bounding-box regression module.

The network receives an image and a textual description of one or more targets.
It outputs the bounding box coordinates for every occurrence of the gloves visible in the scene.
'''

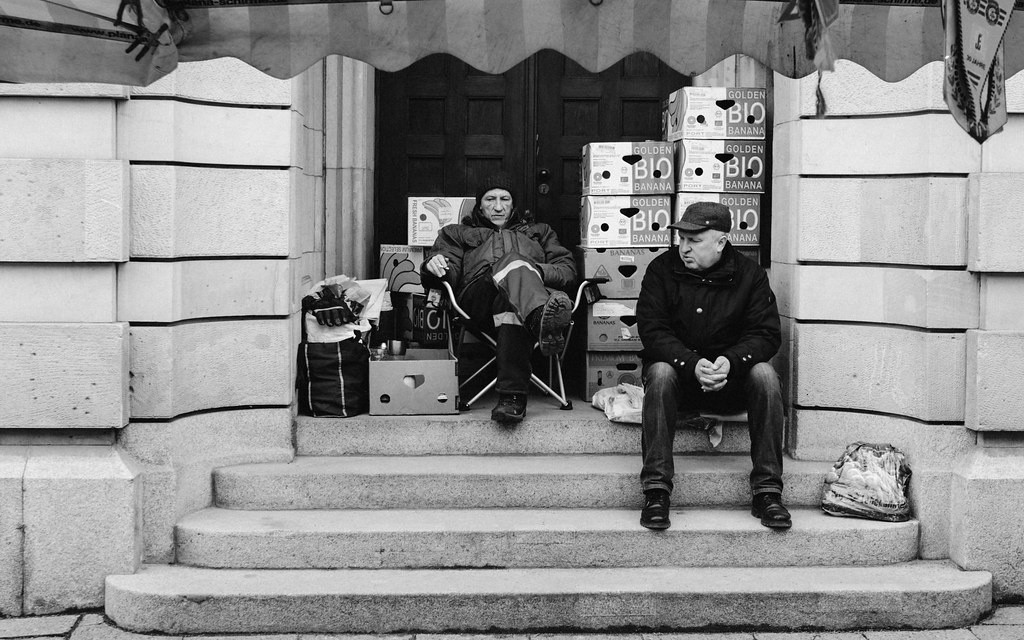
[312,298,356,327]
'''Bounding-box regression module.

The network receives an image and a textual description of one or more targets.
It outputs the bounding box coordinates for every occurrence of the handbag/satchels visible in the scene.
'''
[297,330,371,418]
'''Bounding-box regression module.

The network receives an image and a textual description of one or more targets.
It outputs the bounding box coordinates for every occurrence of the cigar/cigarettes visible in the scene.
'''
[436,264,450,270]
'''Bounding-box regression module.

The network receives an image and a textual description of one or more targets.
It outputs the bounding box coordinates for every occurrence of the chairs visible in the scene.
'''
[675,140,767,192]
[440,273,587,411]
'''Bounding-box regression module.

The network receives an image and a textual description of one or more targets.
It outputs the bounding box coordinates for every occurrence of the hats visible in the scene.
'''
[477,169,518,205]
[667,202,733,233]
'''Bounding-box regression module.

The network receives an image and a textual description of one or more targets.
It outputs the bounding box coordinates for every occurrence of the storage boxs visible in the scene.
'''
[408,197,476,246]
[667,86,768,140]
[581,142,674,196]
[588,300,648,351]
[584,248,668,300]
[380,244,452,293]
[673,194,762,244]
[587,352,643,403]
[579,197,671,248]
[370,349,459,416]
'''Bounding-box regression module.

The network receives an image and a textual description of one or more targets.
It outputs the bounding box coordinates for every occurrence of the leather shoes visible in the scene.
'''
[751,492,792,527]
[640,489,670,529]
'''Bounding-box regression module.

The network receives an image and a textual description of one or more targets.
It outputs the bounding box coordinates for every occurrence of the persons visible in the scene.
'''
[419,171,578,424]
[635,200,793,530]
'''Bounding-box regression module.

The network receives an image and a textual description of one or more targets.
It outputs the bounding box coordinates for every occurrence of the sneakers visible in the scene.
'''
[491,393,526,421]
[532,291,573,356]
